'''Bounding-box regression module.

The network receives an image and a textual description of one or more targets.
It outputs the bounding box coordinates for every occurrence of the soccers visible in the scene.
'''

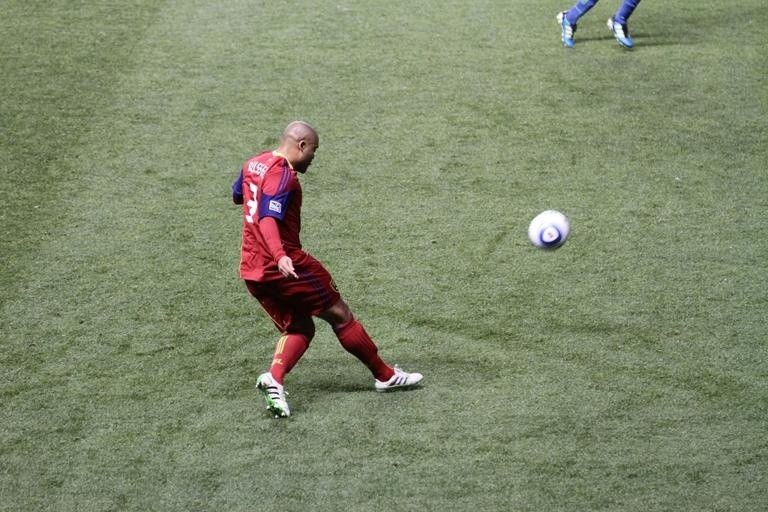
[528,210,569,249]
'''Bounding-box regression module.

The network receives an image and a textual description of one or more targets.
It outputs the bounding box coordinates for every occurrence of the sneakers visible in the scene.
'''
[375,364,424,392]
[556,10,575,48]
[257,372,290,418]
[607,15,633,48]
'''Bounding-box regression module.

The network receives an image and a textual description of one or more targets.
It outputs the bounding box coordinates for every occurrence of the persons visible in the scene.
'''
[233,121,423,418]
[556,0,641,49]
